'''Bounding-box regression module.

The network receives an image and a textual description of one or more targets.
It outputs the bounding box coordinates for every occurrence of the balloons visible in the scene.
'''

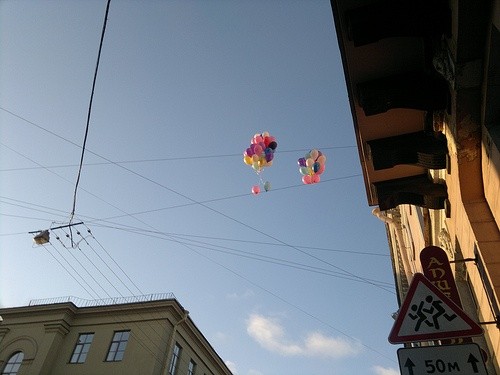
[297,148,327,185]
[243,131,279,195]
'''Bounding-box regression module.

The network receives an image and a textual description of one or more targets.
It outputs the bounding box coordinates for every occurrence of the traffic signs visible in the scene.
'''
[394,344,489,375]
